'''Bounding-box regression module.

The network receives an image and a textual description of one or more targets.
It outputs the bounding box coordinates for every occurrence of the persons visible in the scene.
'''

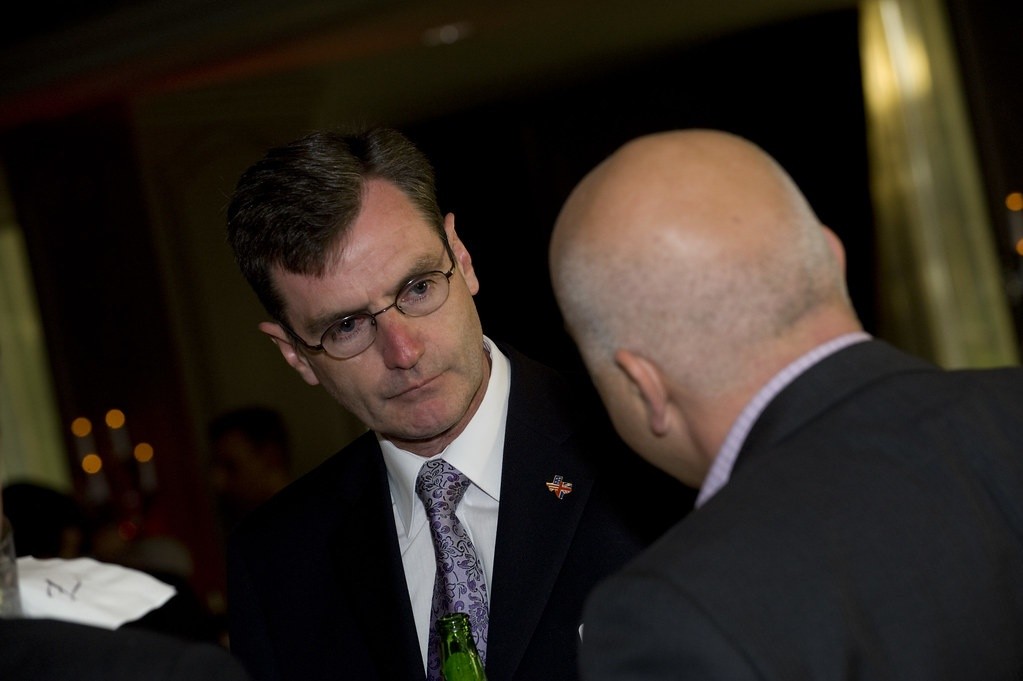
[549,130,1023,681]
[222,117,701,681]
[0,479,249,681]
[205,404,301,564]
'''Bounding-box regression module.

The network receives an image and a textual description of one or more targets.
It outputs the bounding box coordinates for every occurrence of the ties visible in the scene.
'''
[415,458,489,681]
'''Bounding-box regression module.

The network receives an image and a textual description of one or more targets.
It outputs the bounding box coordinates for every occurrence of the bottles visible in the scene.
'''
[435,613,488,681]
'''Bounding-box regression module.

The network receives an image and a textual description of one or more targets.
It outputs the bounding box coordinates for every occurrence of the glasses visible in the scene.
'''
[278,228,456,360]
